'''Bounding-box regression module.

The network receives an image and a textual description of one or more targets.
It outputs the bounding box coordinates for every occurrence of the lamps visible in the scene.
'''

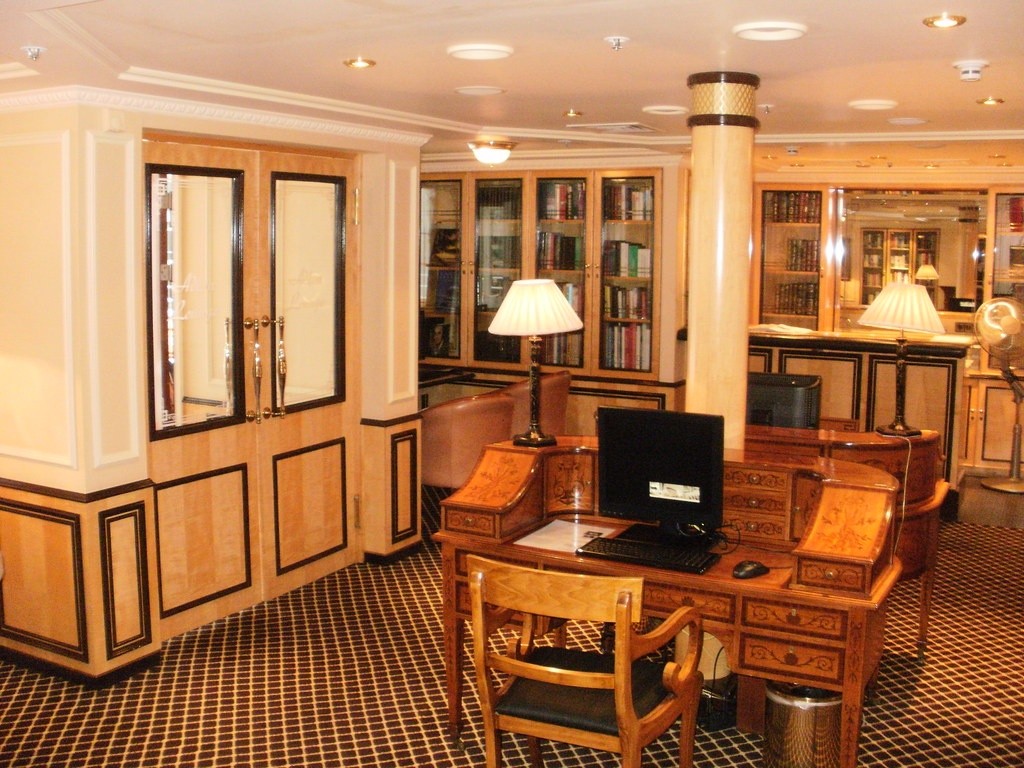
[857,282,946,438]
[489,277,583,447]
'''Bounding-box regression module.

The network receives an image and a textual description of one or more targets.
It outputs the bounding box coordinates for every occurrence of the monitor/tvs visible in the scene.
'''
[420,308,426,360]
[747,372,822,430]
[597,406,724,526]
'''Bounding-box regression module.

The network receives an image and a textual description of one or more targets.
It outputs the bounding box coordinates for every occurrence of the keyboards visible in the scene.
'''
[576,537,720,575]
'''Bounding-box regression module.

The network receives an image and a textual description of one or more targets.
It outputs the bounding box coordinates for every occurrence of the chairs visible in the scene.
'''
[499,369,573,439]
[425,391,514,488]
[465,553,704,768]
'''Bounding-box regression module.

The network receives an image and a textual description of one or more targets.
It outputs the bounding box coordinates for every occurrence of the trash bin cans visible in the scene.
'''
[763,681,843,767]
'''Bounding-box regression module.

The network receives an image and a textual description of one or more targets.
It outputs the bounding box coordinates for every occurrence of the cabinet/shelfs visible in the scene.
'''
[980,186,1024,375]
[420,171,532,373]
[753,182,835,331]
[859,226,941,309]
[528,167,665,380]
[958,378,1024,479]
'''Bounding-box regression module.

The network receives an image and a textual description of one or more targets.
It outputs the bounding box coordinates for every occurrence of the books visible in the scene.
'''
[762,191,823,319]
[428,180,653,374]
[860,231,935,302]
[1009,196,1024,306]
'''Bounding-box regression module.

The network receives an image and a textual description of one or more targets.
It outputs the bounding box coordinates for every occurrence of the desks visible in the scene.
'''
[420,367,476,409]
[430,434,903,768]
[744,416,950,648]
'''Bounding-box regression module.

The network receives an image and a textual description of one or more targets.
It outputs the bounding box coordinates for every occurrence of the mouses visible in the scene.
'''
[733,560,769,579]
[450,368,462,374]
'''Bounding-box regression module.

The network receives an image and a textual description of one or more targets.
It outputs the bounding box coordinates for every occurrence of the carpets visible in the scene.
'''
[953,472,1024,529]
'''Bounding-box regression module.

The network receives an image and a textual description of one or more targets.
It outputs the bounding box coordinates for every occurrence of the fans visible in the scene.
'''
[973,298,1024,494]
